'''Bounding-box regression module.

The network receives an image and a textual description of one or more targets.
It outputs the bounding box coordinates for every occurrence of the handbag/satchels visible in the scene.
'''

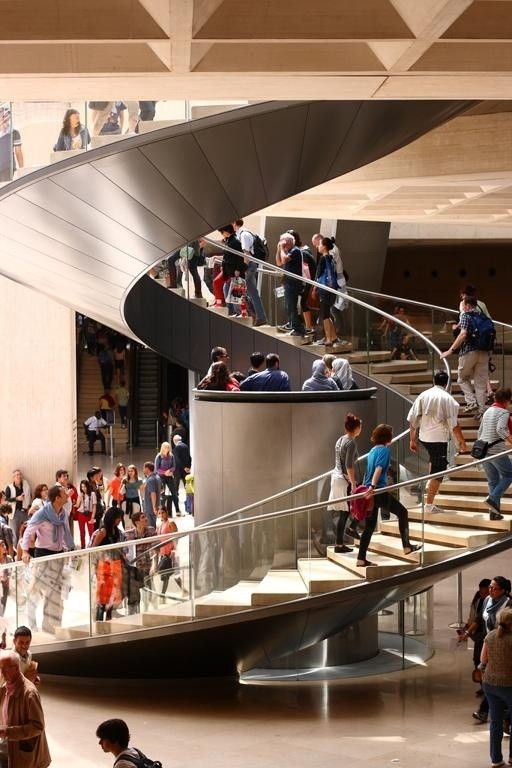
[317,264,336,306]
[472,440,489,466]
[307,284,321,310]
[301,263,311,285]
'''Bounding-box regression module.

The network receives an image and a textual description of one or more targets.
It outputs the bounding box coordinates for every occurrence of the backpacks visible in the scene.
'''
[240,230,269,261]
[114,746,162,768]
[467,312,496,351]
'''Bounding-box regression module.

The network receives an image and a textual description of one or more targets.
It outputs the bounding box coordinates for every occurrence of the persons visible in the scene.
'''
[476,606,512,767]
[1,347,512,649]
[376,303,419,362]
[0,650,53,768]
[52,108,91,151]
[86,101,156,137]
[150,219,348,348]
[457,574,512,738]
[440,283,496,420]
[456,578,491,696]
[0,106,24,182]
[96,718,148,768]
[0,625,41,692]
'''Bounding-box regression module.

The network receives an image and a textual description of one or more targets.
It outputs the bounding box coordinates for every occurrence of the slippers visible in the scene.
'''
[357,560,377,567]
[404,545,423,555]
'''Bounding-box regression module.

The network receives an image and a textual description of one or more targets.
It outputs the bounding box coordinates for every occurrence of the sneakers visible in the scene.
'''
[211,304,268,325]
[278,322,339,346]
[424,503,444,514]
[488,509,505,519]
[473,711,488,722]
[492,760,505,768]
[335,545,353,553]
[484,496,500,514]
[425,479,439,495]
[463,391,494,421]
[345,528,360,539]
